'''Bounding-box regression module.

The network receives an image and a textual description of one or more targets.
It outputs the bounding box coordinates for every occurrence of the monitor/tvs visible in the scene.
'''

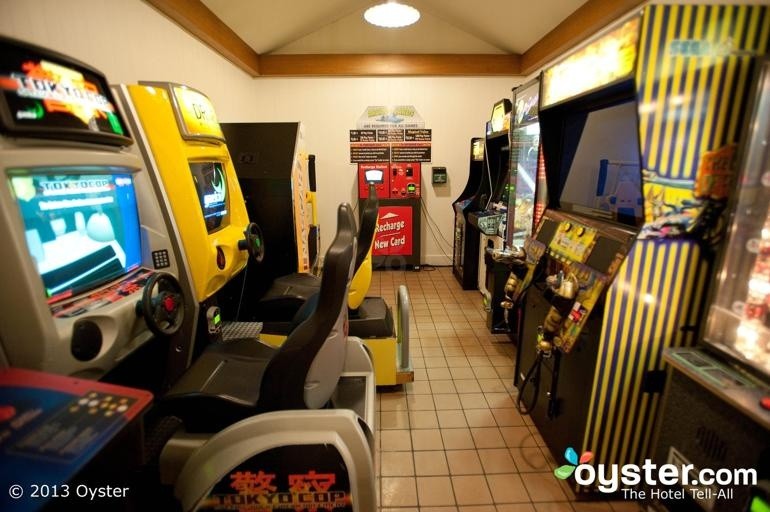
[698,64,770,378]
[10,171,142,306]
[188,161,226,232]
[560,100,643,220]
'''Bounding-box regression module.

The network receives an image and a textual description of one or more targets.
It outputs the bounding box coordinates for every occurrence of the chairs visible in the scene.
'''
[154,197,359,427]
[250,180,382,317]
